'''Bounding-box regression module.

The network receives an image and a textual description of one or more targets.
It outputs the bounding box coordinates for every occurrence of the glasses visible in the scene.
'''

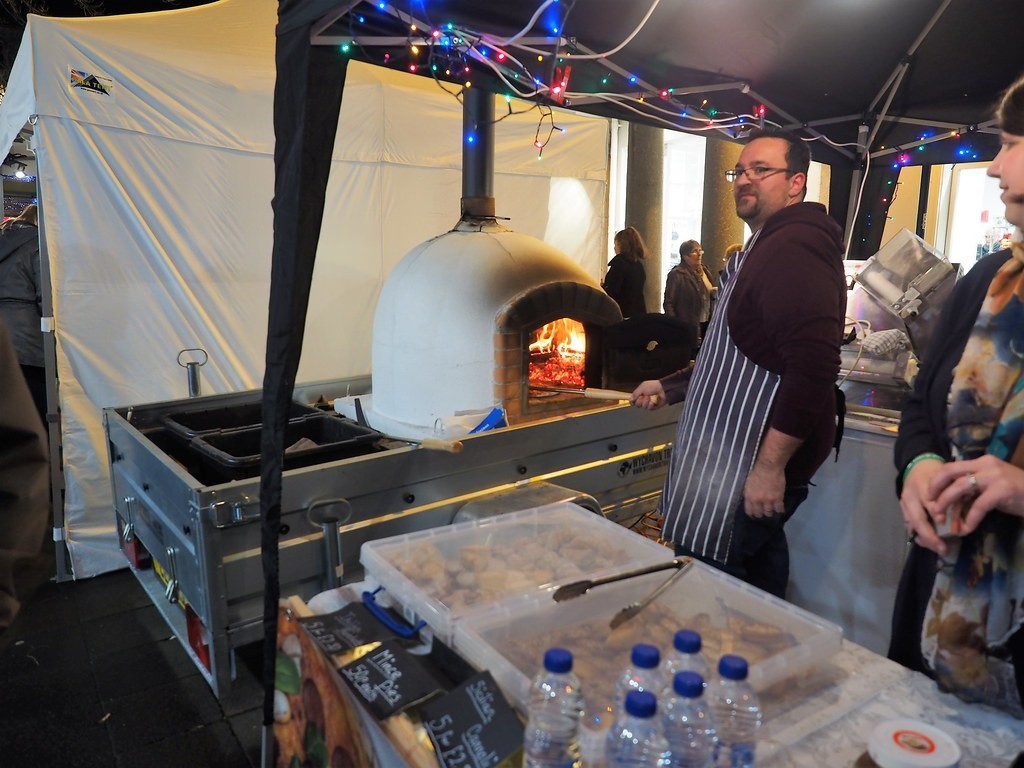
[723,167,797,183]
[690,250,704,255]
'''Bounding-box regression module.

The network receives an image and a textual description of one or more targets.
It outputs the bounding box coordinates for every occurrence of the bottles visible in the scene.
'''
[523,649,583,768]
[658,628,712,681]
[664,670,718,768]
[604,690,666,768]
[710,654,761,768]
[604,644,668,741]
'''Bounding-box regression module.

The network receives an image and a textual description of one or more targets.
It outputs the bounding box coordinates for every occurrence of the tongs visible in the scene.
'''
[552,555,693,630]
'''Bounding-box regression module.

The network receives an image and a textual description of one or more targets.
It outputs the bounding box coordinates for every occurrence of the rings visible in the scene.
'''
[969,473,981,495]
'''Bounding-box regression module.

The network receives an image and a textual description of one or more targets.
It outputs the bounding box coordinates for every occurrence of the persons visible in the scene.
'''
[663,240,716,366]
[888,78,1023,716]
[722,243,744,270]
[630,127,848,600]
[600,227,647,318]
